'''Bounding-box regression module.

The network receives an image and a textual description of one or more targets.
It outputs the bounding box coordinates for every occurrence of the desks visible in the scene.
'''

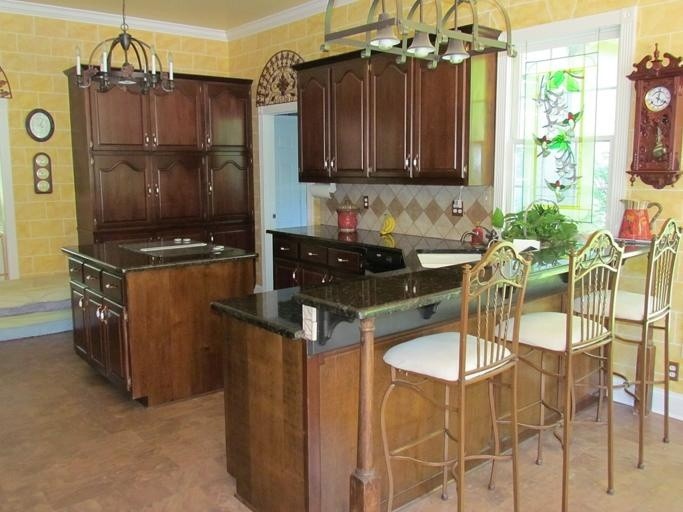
[289,239,658,512]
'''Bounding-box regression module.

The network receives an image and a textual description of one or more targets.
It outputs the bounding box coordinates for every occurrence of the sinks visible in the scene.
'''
[413,248,486,269]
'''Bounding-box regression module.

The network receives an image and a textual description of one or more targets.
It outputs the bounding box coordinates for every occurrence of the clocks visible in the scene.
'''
[25,109,54,142]
[625,39,682,189]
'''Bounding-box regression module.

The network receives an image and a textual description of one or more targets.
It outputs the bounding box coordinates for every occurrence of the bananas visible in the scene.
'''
[379,214,395,235]
[382,235,395,247]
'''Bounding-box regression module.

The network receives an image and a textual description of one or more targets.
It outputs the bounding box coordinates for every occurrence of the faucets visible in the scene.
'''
[460,229,484,244]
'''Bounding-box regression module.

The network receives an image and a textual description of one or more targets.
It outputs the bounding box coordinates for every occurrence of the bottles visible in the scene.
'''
[337,198,360,235]
[472,221,483,246]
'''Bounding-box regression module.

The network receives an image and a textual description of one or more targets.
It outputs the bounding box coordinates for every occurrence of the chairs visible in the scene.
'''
[487,226,622,512]
[563,215,681,470]
[377,239,531,512]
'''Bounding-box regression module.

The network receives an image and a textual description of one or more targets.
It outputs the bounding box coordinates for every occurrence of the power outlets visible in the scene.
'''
[451,200,464,217]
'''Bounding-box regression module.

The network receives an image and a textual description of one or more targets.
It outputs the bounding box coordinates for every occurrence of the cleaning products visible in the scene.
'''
[473,221,485,243]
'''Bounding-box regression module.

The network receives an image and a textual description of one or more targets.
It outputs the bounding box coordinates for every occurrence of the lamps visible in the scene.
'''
[62,0,177,97]
[320,0,517,69]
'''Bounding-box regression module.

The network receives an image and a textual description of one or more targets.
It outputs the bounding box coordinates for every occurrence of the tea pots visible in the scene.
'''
[616,195,664,242]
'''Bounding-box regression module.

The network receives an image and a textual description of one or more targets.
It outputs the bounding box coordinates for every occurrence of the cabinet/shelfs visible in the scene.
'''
[75,230,204,247]
[297,233,366,290]
[206,224,255,252]
[62,64,203,151]
[264,230,299,291]
[290,48,371,184]
[201,74,253,151]
[61,240,261,408]
[71,150,206,234]
[204,151,255,230]
[370,24,501,187]
